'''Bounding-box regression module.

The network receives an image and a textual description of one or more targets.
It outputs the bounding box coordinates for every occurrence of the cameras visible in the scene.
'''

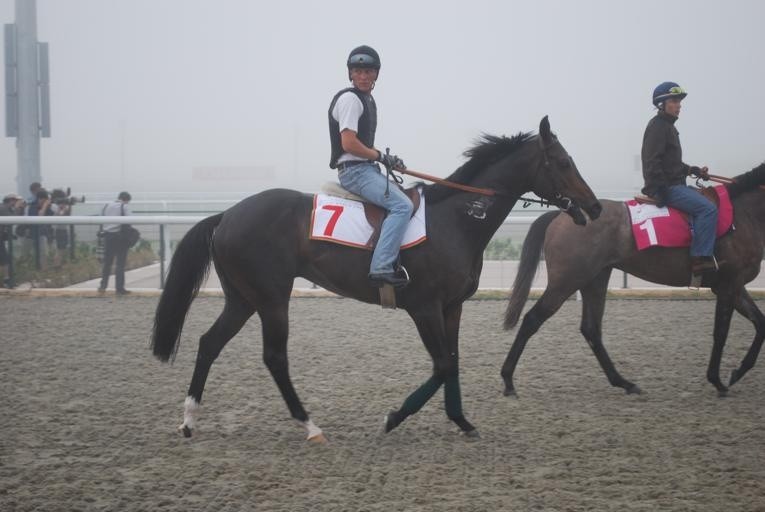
[11,199,33,211]
[69,195,86,203]
[52,197,77,205]
[41,186,71,197]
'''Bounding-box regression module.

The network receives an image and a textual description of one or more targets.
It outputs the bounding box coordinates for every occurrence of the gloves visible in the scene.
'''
[690,165,703,177]
[392,155,406,173]
[655,190,667,207]
[378,151,397,166]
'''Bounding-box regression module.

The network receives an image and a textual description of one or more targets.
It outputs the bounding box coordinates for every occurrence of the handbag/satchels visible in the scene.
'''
[118,204,140,248]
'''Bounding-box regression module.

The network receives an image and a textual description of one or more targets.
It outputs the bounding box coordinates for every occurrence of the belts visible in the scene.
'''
[336,160,357,170]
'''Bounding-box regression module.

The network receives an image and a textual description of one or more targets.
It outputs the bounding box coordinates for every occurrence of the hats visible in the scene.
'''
[6,192,22,199]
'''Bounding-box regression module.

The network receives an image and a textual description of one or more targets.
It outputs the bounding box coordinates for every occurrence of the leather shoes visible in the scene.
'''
[98,287,104,292]
[116,289,132,295]
[370,274,409,288]
[693,259,726,276]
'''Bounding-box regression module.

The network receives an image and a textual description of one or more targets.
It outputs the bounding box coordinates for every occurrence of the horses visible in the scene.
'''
[501,161,765,402]
[149,115,603,445]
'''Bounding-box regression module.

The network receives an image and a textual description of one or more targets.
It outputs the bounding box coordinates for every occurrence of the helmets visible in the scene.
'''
[653,81,687,103]
[347,45,381,68]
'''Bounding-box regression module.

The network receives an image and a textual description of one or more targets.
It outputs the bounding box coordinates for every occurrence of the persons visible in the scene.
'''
[642,83,726,273]
[329,45,418,290]
[0,196,25,288]
[25,183,43,279]
[96,192,131,296]
[28,190,58,276]
[48,189,69,265]
